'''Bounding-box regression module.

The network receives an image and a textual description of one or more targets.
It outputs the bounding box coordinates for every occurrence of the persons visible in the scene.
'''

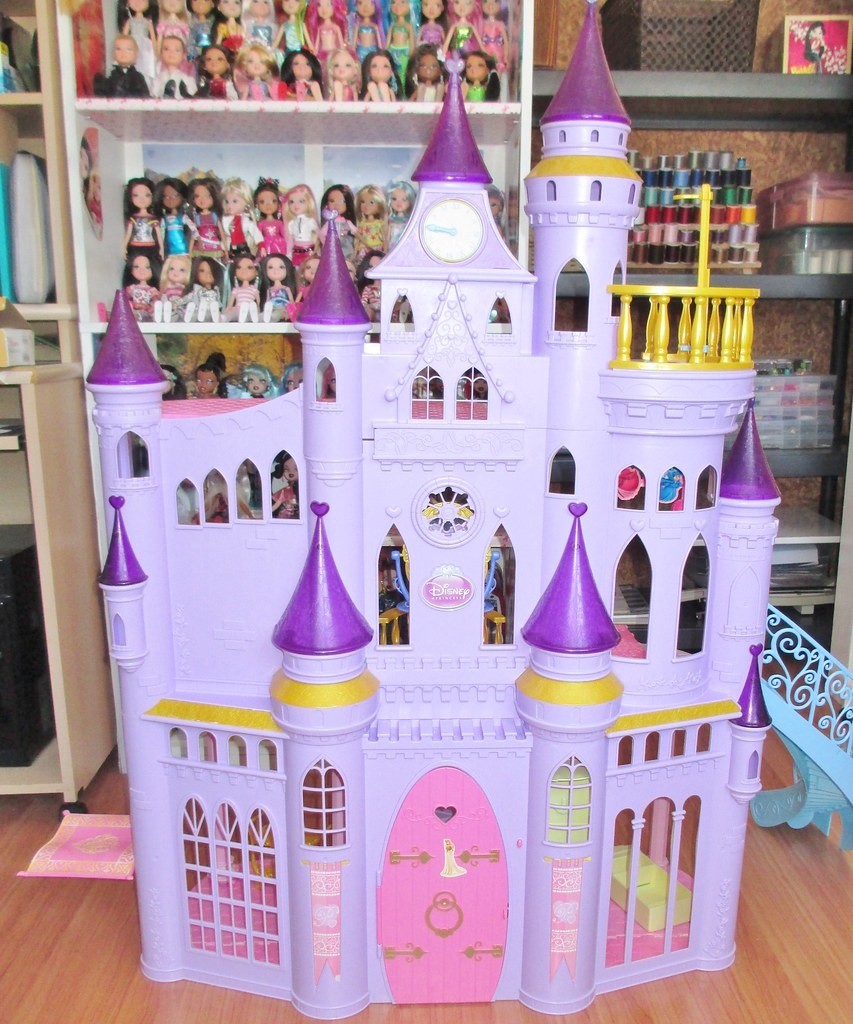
[100,0,512,646]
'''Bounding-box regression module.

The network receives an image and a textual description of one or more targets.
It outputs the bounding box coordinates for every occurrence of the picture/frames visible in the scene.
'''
[783,14,853,74]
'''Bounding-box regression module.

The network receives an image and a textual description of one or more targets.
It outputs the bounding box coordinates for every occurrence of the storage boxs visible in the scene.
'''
[755,173,853,275]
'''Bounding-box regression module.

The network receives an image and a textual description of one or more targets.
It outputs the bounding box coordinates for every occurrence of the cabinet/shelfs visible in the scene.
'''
[532,69,853,707]
[0,0,116,817]
[52,0,534,576]
